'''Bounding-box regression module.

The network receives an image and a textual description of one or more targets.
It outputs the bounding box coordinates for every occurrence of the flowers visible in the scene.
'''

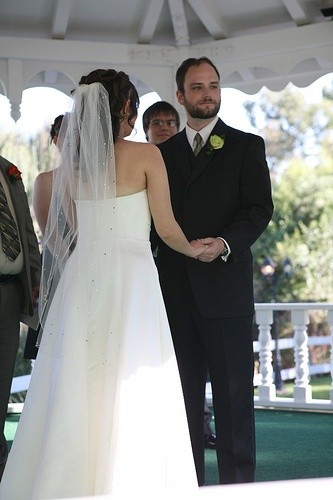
[7,165,23,184]
[206,135,224,155]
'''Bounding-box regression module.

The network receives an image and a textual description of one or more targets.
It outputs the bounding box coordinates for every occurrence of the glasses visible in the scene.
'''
[150,119,176,127]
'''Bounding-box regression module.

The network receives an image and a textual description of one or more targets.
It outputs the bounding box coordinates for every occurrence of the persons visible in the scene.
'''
[0,69,209,500]
[0,157,43,477]
[146,58,274,487]
[22,114,81,359]
[142,101,216,448]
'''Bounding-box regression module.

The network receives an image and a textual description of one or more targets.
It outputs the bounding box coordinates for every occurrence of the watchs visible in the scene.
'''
[219,239,229,257]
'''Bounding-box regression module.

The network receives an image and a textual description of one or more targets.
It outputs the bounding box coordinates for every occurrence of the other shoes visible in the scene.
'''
[203,433,216,449]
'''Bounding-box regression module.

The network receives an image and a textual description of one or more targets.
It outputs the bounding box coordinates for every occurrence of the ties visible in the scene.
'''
[0,181,21,263]
[194,133,202,158]
